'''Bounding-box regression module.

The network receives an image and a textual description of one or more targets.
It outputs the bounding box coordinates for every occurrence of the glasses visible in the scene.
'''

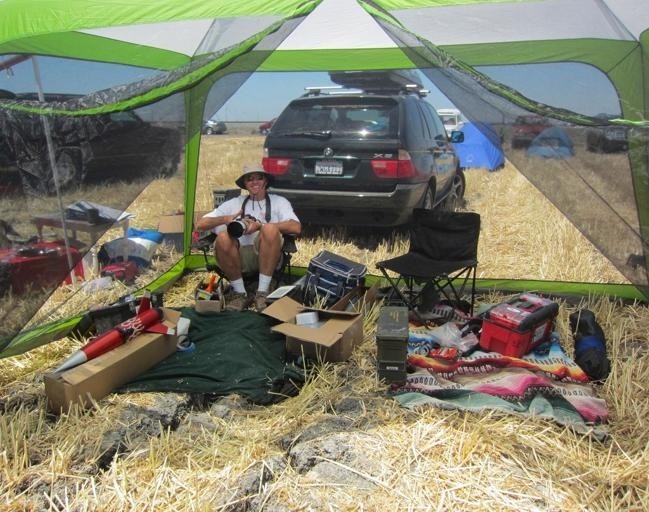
[242,173,265,182]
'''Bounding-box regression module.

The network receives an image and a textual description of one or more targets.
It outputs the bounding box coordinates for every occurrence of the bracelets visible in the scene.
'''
[257,222,263,231]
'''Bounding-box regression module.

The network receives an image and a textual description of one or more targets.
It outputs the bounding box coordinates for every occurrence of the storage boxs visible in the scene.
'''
[44,304,187,418]
[372,301,413,387]
[261,297,365,366]
[479,289,558,358]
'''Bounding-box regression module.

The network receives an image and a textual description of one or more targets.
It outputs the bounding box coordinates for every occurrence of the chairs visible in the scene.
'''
[378,208,478,328]
[194,233,297,299]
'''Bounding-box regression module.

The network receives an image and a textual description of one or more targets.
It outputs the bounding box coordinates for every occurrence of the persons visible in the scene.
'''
[194,160,301,312]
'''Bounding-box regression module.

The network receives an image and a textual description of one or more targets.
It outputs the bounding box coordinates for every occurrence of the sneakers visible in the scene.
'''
[226,292,249,311]
[253,291,269,313]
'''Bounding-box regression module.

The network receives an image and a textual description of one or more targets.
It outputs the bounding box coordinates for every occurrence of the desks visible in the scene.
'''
[33,212,128,269]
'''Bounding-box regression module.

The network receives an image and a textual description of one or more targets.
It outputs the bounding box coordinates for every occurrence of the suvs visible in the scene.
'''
[262,69,465,227]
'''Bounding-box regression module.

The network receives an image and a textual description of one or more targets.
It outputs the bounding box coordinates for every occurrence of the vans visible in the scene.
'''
[436,109,464,138]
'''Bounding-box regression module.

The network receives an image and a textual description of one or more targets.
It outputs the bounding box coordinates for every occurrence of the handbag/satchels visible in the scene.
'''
[304,248,367,303]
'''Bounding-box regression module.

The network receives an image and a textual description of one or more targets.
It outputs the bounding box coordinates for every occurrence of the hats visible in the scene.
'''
[235,164,275,190]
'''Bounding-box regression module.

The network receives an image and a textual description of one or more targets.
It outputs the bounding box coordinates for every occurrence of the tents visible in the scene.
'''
[451,118,507,172]
[526,125,577,160]
[0,0,649,364]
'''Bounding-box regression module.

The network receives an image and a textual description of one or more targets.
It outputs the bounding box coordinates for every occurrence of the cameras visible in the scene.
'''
[227,214,261,238]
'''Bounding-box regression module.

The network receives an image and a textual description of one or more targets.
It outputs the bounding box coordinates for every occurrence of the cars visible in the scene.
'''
[584,113,626,153]
[0,93,181,193]
[511,115,550,148]
[260,118,277,134]
[202,120,226,134]
